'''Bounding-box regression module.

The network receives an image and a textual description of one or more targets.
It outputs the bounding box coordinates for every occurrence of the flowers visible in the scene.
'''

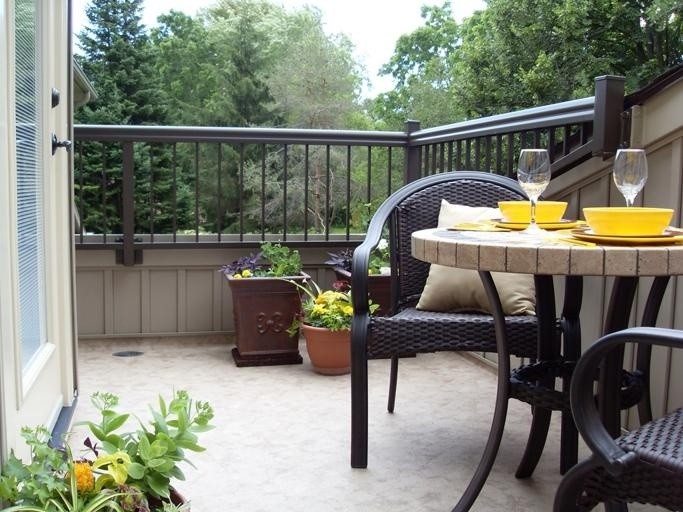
[219,234,388,328]
[0,424,143,512]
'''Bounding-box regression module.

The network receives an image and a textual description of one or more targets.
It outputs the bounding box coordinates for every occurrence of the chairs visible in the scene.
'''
[350,170,589,473]
[552,326,683,512]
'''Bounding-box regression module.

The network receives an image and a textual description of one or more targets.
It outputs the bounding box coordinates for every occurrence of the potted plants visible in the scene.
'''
[84,388,190,512]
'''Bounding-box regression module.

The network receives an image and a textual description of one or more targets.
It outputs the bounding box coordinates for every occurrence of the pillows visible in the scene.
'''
[416,199,540,315]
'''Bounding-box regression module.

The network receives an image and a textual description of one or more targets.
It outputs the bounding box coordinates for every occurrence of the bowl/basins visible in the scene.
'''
[582,207,674,236]
[498,200,568,224]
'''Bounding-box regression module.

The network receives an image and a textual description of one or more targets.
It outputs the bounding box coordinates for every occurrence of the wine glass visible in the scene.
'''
[612,149,649,208]
[517,149,551,236]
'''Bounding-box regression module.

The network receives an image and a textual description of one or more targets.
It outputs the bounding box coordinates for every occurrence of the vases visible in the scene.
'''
[225,270,387,376]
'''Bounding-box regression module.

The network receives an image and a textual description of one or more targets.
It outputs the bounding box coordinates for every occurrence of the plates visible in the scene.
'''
[490,220,578,229]
[572,229,683,245]
